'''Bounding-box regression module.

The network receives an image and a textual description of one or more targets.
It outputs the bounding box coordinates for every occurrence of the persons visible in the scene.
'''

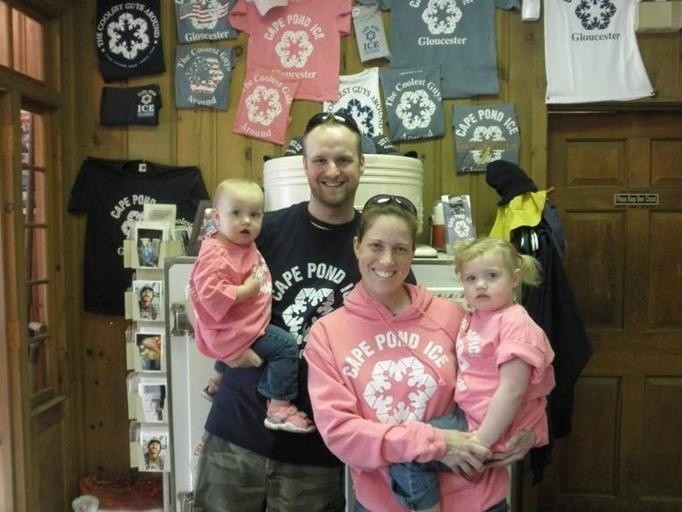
[194,106,420,512]
[385,235,558,512]
[142,437,166,470]
[188,174,319,437]
[138,284,161,318]
[300,195,548,512]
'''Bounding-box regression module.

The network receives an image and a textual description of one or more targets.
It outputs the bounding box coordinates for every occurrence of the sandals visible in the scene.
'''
[201,377,220,399]
[264,404,314,434]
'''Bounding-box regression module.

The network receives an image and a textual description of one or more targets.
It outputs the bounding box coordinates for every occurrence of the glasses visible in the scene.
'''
[362,195,417,214]
[303,113,359,134]
